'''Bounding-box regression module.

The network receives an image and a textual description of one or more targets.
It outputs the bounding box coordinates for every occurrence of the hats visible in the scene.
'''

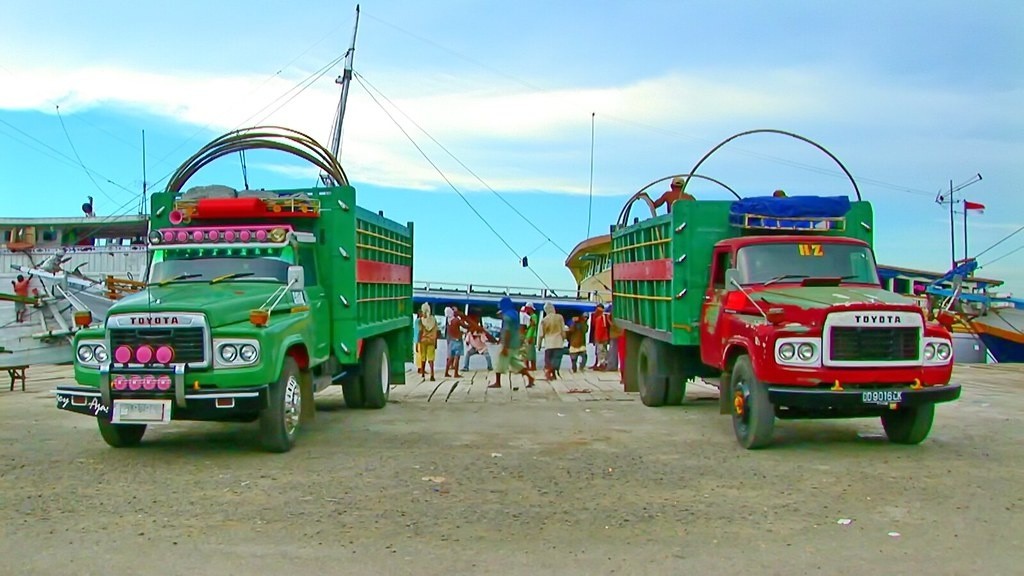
[673,177,684,186]
[525,302,536,310]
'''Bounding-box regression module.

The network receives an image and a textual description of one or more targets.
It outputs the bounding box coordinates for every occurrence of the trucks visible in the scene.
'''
[50,183,414,454]
[608,196,961,449]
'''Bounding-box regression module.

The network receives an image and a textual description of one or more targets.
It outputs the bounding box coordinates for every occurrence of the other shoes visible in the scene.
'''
[445,375,452,377]
[488,383,501,388]
[430,376,435,381]
[488,368,493,370]
[588,366,597,369]
[593,366,605,371]
[454,375,464,378]
[460,367,469,371]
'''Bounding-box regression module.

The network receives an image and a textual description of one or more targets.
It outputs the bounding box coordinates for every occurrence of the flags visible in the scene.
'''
[965,202,985,209]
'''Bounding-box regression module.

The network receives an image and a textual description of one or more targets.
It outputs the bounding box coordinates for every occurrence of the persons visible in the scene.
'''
[636,177,696,214]
[43,249,65,273]
[414,297,618,387]
[11,274,33,323]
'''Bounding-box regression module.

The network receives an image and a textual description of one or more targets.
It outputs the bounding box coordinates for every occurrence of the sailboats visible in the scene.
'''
[914,224,1024,363]
